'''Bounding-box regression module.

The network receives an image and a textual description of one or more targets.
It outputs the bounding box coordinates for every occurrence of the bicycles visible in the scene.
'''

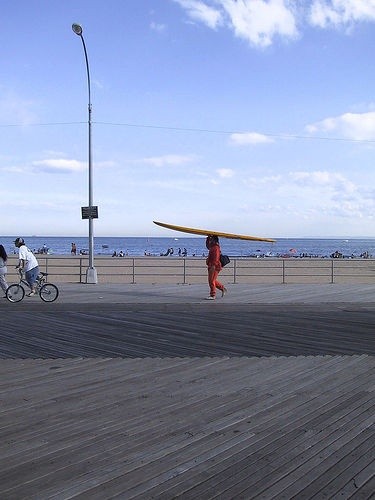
[5,266,58,303]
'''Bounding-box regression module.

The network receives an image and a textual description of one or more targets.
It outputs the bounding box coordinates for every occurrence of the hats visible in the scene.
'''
[12,237,24,243]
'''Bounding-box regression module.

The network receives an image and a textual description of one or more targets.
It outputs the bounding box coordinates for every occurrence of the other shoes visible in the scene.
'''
[220,288,227,297]
[204,296,215,300]
[3,295,12,299]
[28,292,35,296]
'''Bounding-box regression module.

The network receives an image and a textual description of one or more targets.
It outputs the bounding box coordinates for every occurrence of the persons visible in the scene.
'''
[330,250,342,258]
[112,250,124,257]
[144,250,147,255]
[170,247,187,257]
[0,244,11,298]
[71,243,86,255]
[12,237,40,297]
[205,235,227,300]
[31,244,47,254]
[360,251,372,259]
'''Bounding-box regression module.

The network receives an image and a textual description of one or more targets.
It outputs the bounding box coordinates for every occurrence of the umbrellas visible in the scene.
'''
[289,249,296,256]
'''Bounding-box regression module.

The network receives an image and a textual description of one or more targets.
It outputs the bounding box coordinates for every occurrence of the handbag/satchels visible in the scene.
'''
[219,254,230,267]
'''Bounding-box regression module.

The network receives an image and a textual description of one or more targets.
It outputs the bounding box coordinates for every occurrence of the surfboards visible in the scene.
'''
[153,220,276,242]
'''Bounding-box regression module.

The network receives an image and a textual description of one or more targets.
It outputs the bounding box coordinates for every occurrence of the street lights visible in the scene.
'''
[70,22,98,284]
